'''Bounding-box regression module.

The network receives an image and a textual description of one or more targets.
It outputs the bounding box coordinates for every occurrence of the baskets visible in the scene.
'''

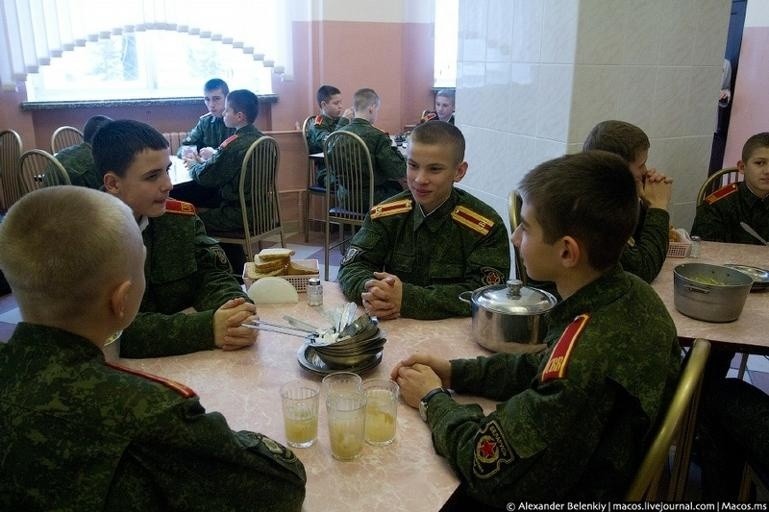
[242,260,319,293]
[666,229,690,257]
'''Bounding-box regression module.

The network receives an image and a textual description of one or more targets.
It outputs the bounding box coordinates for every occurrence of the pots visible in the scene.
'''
[459,278,559,355]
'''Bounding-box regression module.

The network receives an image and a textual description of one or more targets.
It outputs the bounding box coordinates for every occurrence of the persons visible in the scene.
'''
[392,149,719,512]
[523,121,673,304]
[680,132,769,382]
[175,79,454,284]
[43,115,113,188]
[92,119,260,358]
[1,184,306,512]
[336,120,510,321]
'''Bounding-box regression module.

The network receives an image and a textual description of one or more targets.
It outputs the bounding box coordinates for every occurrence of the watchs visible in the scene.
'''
[418,387,454,423]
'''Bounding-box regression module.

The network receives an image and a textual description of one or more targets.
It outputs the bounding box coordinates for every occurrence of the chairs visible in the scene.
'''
[319,124,378,277]
[1,127,26,214]
[300,112,356,254]
[505,187,542,290]
[211,132,286,263]
[696,165,748,203]
[49,124,83,154]
[15,148,70,198]
[629,336,713,505]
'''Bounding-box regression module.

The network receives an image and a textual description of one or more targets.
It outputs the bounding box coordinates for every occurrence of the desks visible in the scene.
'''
[306,134,418,166]
[164,150,198,191]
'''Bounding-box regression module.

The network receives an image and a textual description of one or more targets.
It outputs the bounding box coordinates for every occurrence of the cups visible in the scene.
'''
[181,145,196,158]
[325,369,368,464]
[360,376,400,448]
[277,380,320,449]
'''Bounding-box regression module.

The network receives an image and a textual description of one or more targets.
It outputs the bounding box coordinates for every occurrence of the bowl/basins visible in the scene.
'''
[672,260,751,324]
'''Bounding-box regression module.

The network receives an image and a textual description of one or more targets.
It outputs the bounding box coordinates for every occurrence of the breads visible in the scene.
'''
[245,249,319,279]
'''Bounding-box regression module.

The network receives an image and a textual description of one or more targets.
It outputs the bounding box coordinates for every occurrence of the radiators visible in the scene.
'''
[156,130,194,156]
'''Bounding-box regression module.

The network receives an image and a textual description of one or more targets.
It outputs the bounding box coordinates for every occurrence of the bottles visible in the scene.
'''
[690,236,701,257]
[307,277,324,307]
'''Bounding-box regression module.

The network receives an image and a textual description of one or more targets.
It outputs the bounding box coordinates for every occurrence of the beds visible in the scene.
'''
[118,268,504,512]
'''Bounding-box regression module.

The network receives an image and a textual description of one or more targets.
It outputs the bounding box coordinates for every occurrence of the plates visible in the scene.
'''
[298,343,383,382]
[723,263,769,291]
